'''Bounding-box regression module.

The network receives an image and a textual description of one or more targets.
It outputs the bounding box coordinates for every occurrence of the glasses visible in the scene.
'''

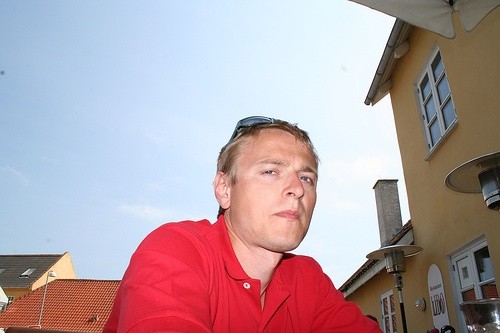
[230,116,275,140]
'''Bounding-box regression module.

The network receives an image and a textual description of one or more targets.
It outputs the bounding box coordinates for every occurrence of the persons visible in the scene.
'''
[101,116,384,333]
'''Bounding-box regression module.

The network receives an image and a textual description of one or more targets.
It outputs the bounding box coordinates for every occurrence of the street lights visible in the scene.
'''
[38,270,57,324]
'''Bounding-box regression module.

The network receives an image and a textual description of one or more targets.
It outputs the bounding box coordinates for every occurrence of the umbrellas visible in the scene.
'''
[348,0,500,39]
